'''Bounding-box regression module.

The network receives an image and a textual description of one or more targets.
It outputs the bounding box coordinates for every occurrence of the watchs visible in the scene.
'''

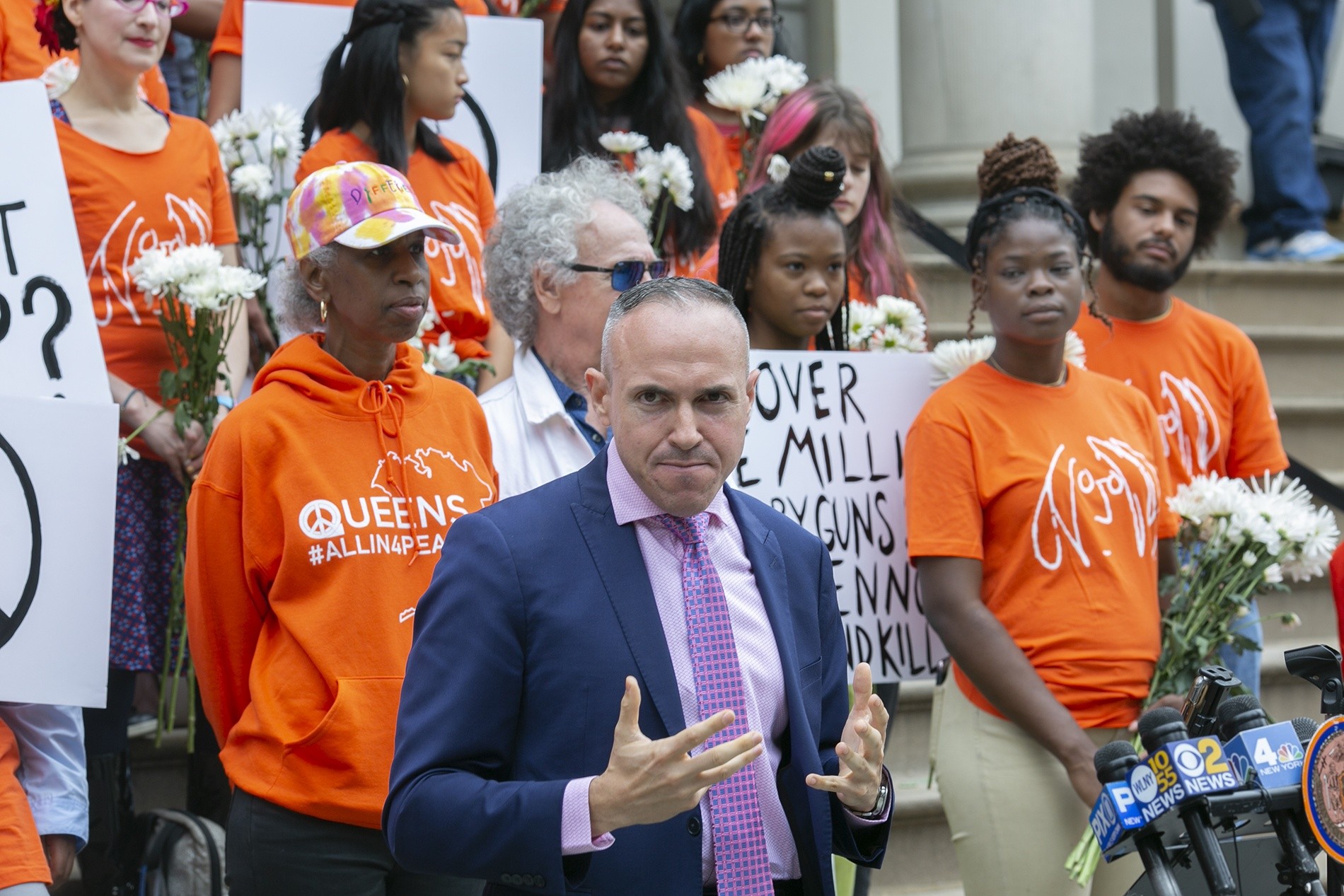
[852,775,888,818]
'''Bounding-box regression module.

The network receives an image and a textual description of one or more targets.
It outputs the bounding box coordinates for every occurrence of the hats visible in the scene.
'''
[284,161,462,262]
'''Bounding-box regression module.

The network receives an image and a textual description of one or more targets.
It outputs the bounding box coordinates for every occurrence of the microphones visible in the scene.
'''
[1124,706,1240,896]
[1089,740,1183,896]
[1290,716,1319,754]
[1216,694,1326,896]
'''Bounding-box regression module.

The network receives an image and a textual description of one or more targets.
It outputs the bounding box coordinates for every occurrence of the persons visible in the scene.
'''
[1207,0,1344,264]
[0,0,1289,896]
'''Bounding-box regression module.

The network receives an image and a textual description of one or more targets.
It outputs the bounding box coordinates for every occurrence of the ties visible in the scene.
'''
[658,515,775,896]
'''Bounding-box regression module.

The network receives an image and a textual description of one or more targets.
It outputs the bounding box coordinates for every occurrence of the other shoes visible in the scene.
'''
[1275,228,1344,264]
[1244,235,1287,262]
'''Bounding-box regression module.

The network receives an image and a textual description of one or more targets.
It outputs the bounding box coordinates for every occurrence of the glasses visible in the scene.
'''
[706,8,784,35]
[511,250,672,293]
[117,0,174,16]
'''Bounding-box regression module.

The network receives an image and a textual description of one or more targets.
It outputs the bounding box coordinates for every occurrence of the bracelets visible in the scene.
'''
[121,388,137,416]
[217,395,237,409]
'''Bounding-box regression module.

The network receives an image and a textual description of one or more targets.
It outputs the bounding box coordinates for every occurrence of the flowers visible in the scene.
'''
[932,327,1084,379]
[697,50,811,190]
[603,131,700,258]
[206,106,299,348]
[1064,466,1341,886]
[113,244,270,753]
[837,290,927,357]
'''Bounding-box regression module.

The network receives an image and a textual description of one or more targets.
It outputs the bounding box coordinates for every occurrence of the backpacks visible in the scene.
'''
[120,808,228,896]
